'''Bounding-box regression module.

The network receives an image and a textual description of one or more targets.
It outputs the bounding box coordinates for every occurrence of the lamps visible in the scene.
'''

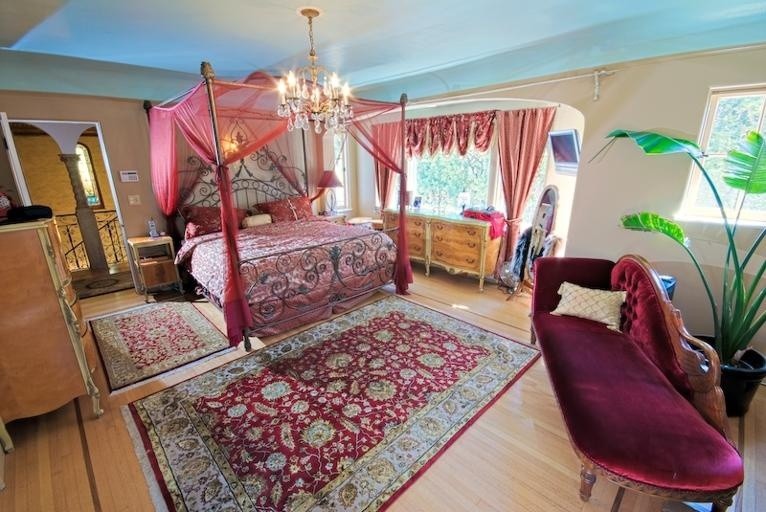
[317,170,344,215]
[273,7,354,135]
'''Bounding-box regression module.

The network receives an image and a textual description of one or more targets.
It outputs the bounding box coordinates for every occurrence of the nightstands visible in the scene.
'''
[127,234,184,303]
[316,214,346,226]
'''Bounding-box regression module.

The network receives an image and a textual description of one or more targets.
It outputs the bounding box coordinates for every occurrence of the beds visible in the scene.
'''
[143,60,414,352]
[530,253,744,511]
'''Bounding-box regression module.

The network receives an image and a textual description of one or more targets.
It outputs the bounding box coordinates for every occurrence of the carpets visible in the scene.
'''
[86,295,239,398]
[120,289,543,512]
[72,271,135,299]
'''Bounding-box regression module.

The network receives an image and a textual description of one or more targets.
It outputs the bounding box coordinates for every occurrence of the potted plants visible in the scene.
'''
[590,127,765,419]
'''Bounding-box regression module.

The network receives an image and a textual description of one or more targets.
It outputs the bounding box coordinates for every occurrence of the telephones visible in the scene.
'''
[148,216,159,238]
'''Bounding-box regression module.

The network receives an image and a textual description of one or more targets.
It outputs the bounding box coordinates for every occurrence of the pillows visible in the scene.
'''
[241,213,271,228]
[181,205,251,237]
[252,197,314,224]
[549,281,626,332]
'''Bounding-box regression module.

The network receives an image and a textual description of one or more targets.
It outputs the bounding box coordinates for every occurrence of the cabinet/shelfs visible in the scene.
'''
[382,209,502,291]
[0,204,105,455]
